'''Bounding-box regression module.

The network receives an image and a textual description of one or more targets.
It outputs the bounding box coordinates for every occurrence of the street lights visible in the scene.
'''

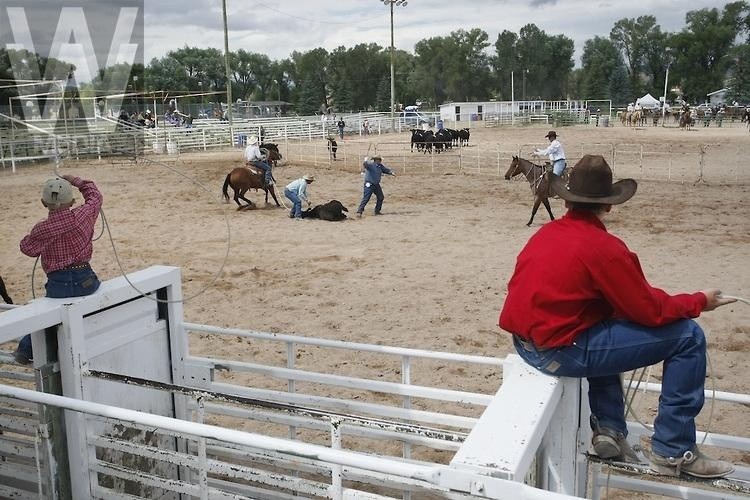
[134,76,140,114]
[382,0,407,130]
[198,81,203,113]
[274,80,280,107]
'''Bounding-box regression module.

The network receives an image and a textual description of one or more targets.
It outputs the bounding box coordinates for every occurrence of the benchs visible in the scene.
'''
[0,113,406,167]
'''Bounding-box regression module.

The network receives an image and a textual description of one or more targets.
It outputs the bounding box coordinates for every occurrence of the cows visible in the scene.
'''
[410,127,470,155]
[300,199,351,222]
[326,138,338,161]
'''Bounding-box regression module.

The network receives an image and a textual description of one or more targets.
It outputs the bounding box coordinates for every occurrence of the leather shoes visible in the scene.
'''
[639,434,734,478]
[590,414,650,473]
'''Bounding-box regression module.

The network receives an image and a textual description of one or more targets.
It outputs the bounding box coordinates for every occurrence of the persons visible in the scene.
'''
[584,99,750,126]
[258,126,264,141]
[244,132,274,186]
[530,131,566,199]
[284,173,317,221]
[321,113,373,161]
[238,127,247,148]
[115,99,228,130]
[13,174,103,365]
[499,154,737,478]
[356,155,396,218]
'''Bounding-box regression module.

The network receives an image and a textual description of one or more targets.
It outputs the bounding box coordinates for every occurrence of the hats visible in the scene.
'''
[247,135,258,144]
[550,154,637,205]
[303,172,316,181]
[372,153,385,159]
[545,130,559,138]
[40,176,74,205]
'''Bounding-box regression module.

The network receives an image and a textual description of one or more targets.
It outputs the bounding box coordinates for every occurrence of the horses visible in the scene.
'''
[221,143,283,208]
[619,103,750,131]
[504,154,574,227]
